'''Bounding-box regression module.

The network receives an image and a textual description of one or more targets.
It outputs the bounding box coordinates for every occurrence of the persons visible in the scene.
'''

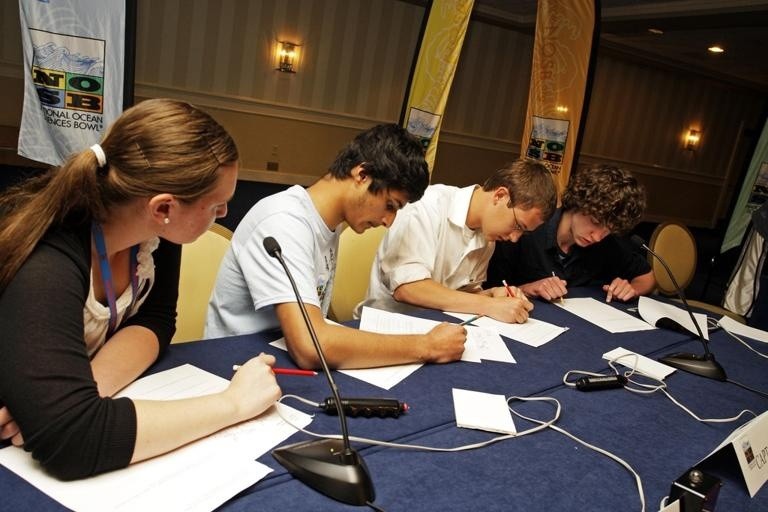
[364,155,559,326]
[199,119,467,370]
[0,91,283,481]
[479,160,658,305]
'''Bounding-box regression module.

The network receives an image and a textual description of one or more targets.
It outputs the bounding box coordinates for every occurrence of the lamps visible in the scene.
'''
[682,126,703,153]
[271,37,302,74]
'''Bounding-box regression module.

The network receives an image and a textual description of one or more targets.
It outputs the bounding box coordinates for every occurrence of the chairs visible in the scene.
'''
[172,223,235,344]
[329,221,387,323]
[648,220,746,322]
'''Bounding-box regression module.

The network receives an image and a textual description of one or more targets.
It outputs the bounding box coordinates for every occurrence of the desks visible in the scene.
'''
[0,290,768,512]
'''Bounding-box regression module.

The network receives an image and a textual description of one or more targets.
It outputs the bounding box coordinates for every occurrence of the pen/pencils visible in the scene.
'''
[502,279,513,297]
[232,364,318,376]
[459,314,485,325]
[552,271,564,304]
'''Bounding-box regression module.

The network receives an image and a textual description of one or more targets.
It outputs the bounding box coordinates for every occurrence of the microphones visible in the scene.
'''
[631,233,728,383]
[263,235,377,505]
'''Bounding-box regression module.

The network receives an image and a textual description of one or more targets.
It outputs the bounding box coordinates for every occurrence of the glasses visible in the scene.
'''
[511,208,530,237]
